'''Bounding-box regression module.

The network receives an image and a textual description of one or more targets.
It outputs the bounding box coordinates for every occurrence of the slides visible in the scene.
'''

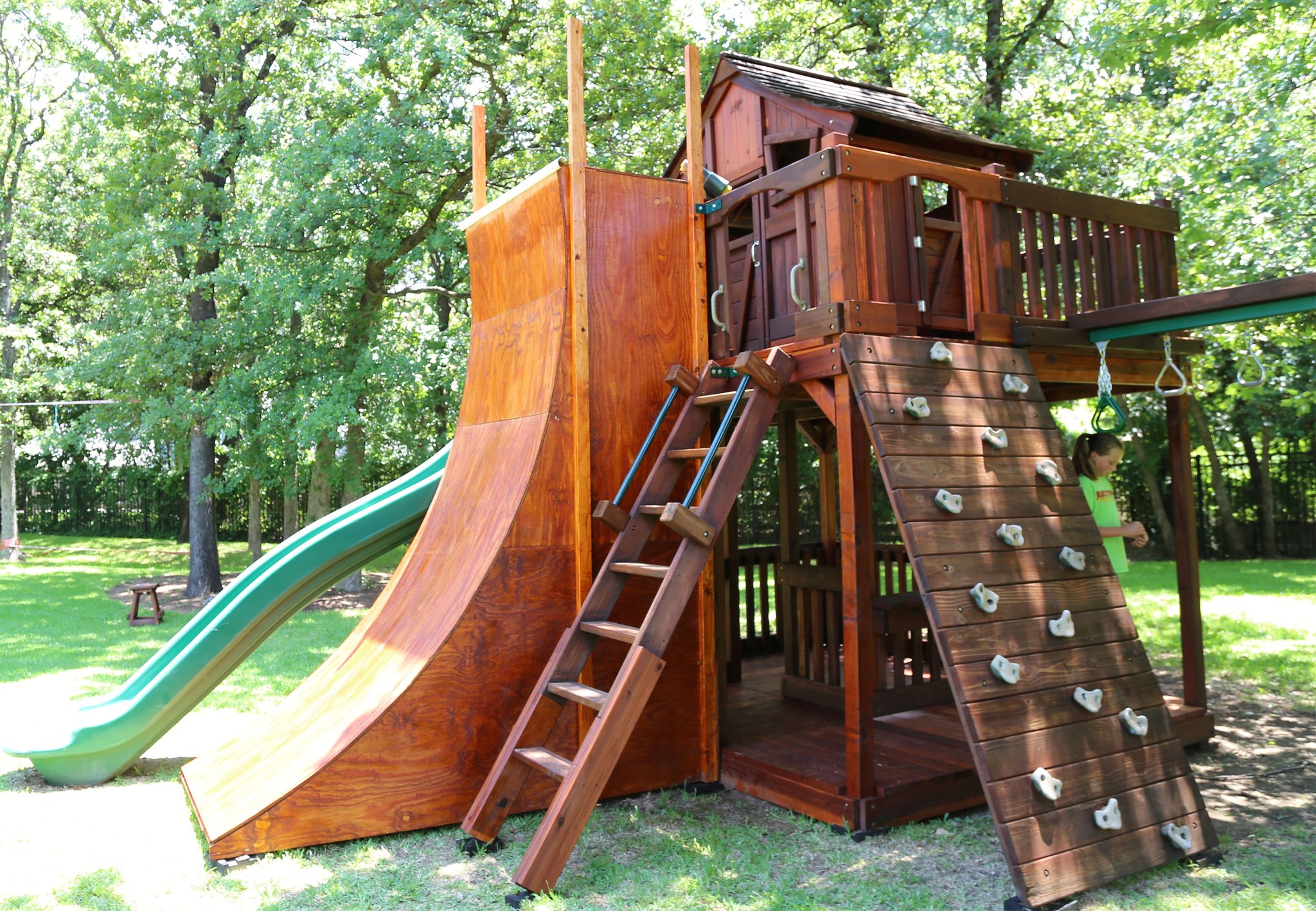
[5,434,453,789]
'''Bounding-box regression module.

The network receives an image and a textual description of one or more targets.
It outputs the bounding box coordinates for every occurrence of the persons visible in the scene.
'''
[1072,433,1149,574]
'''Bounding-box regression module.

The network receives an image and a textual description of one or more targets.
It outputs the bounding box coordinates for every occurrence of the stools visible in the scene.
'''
[127,583,165,626]
